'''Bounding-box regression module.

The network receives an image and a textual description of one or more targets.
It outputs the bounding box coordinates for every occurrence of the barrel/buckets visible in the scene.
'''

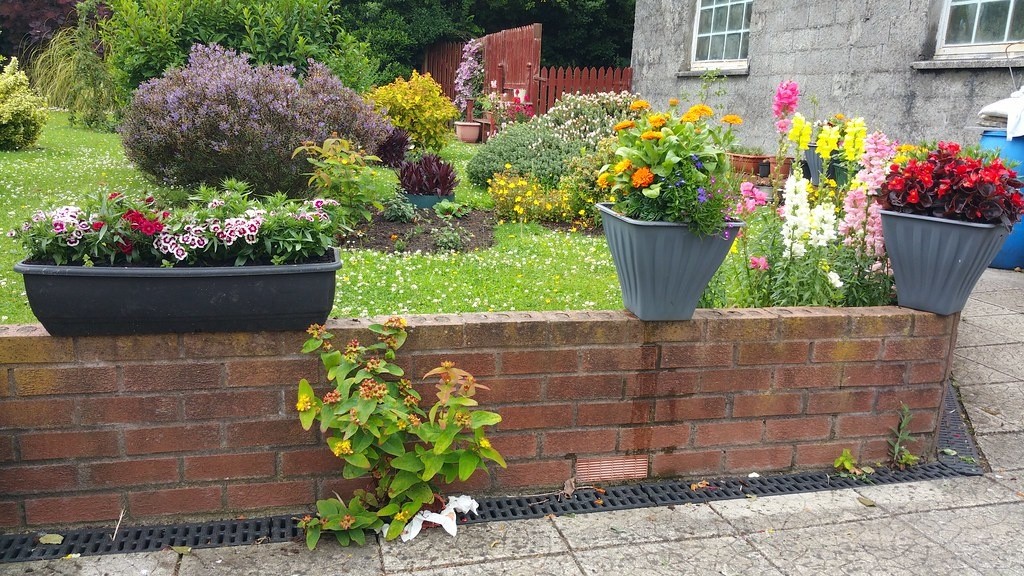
[976,128,1024,270]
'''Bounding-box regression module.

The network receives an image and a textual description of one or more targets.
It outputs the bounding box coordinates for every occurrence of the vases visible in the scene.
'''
[454,121,482,143]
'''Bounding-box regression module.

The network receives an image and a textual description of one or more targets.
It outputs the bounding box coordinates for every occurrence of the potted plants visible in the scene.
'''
[832,117,868,189]
[804,118,828,186]
[593,98,747,322]
[393,151,461,209]
[724,145,770,175]
[770,80,801,179]
[6,174,356,334]
[873,138,1024,316]
[482,92,497,121]
[758,158,771,177]
[787,112,811,181]
[814,114,852,180]
